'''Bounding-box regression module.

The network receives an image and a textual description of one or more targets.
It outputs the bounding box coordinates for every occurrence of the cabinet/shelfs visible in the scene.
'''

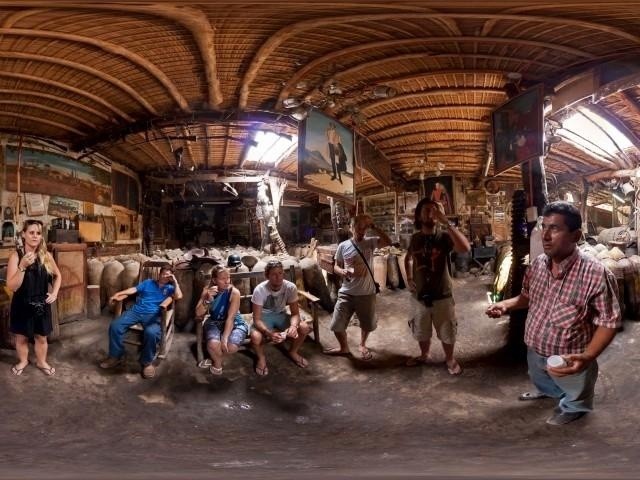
[47,244,89,323]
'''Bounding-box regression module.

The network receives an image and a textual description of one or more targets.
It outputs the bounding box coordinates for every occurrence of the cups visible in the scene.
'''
[547,354,567,370]
[280,332,286,340]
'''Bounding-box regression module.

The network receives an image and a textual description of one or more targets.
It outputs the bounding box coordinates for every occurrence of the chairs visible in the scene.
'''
[115,260,176,359]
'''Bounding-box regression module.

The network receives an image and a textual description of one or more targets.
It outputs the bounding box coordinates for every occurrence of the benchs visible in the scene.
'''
[195,269,321,363]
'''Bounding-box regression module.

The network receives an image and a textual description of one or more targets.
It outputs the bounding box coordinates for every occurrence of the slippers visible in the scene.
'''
[255,364,269,377]
[10,360,30,376]
[361,351,372,361]
[322,348,351,356]
[198,359,212,368]
[209,364,223,376]
[289,355,308,368]
[446,361,463,376]
[407,356,432,367]
[35,362,55,375]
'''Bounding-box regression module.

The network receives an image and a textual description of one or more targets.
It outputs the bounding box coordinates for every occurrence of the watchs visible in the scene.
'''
[291,324,300,329]
[447,223,452,229]
[17,266,27,272]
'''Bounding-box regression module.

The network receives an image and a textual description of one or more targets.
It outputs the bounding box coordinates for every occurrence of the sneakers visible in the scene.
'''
[99,357,124,370]
[519,390,547,400]
[138,364,155,379]
[547,407,586,425]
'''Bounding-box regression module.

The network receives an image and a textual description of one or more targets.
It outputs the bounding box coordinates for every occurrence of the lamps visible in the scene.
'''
[405,130,445,181]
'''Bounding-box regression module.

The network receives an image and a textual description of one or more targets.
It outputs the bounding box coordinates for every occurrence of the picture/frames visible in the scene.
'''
[299,110,356,205]
[491,81,543,177]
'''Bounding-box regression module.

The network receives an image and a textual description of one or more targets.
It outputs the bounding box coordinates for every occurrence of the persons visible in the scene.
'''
[320,215,393,362]
[100,265,183,379]
[248,260,310,377]
[6,220,61,377]
[404,198,470,377]
[326,121,344,185]
[485,200,622,426]
[430,183,447,212]
[194,265,248,375]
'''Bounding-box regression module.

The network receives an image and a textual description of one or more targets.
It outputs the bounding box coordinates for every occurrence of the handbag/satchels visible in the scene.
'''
[350,238,380,296]
